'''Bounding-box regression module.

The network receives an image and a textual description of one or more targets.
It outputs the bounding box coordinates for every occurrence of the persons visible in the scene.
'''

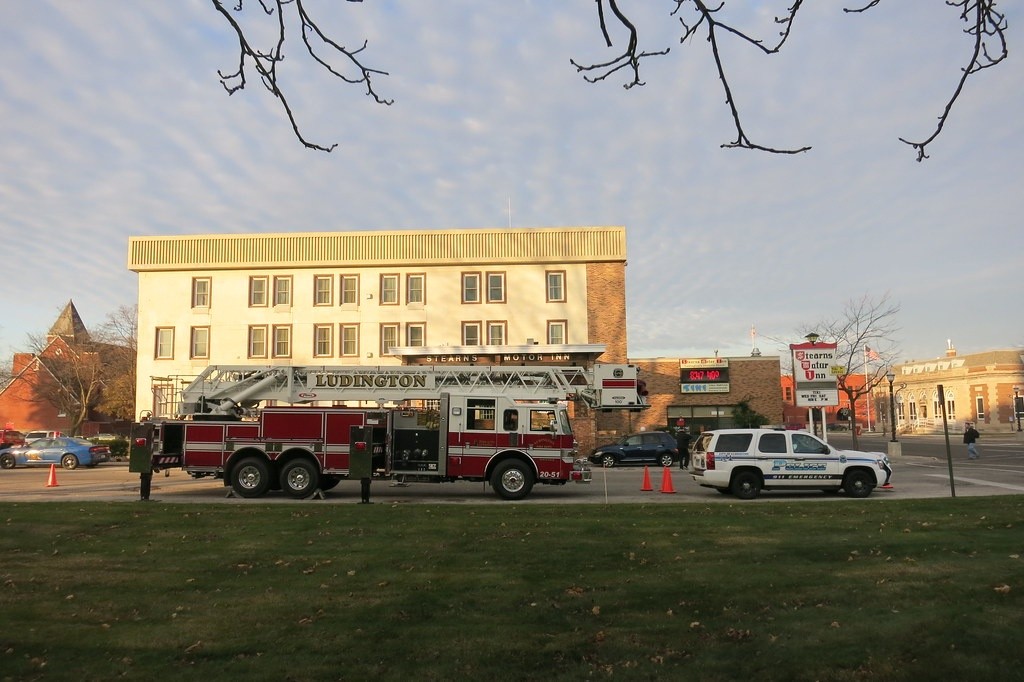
[675,431,694,471]
[963,423,980,459]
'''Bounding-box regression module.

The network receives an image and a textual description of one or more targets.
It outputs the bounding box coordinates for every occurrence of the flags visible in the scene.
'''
[865,346,880,361]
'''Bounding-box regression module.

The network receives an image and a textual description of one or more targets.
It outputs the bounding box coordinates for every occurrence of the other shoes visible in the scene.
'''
[683,465,688,470]
[680,468,683,470]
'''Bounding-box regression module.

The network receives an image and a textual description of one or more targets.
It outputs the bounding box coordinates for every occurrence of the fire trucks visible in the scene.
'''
[124,363,594,500]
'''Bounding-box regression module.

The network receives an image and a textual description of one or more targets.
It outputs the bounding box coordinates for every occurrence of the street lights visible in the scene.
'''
[1013,384,1023,431]
[886,369,900,441]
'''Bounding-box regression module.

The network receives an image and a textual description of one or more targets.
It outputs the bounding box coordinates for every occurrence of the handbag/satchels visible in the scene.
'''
[970,428,979,438]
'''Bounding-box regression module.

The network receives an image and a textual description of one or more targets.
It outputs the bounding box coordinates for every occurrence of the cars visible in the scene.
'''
[24,430,69,446]
[0,437,112,470]
[0,430,26,448]
[86,433,124,441]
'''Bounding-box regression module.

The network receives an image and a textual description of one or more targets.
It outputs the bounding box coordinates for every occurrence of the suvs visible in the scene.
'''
[587,430,679,467]
[689,428,892,500]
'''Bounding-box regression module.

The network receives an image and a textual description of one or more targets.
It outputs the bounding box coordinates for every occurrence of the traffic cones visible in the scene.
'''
[657,466,677,493]
[640,467,654,491]
[44,464,60,487]
